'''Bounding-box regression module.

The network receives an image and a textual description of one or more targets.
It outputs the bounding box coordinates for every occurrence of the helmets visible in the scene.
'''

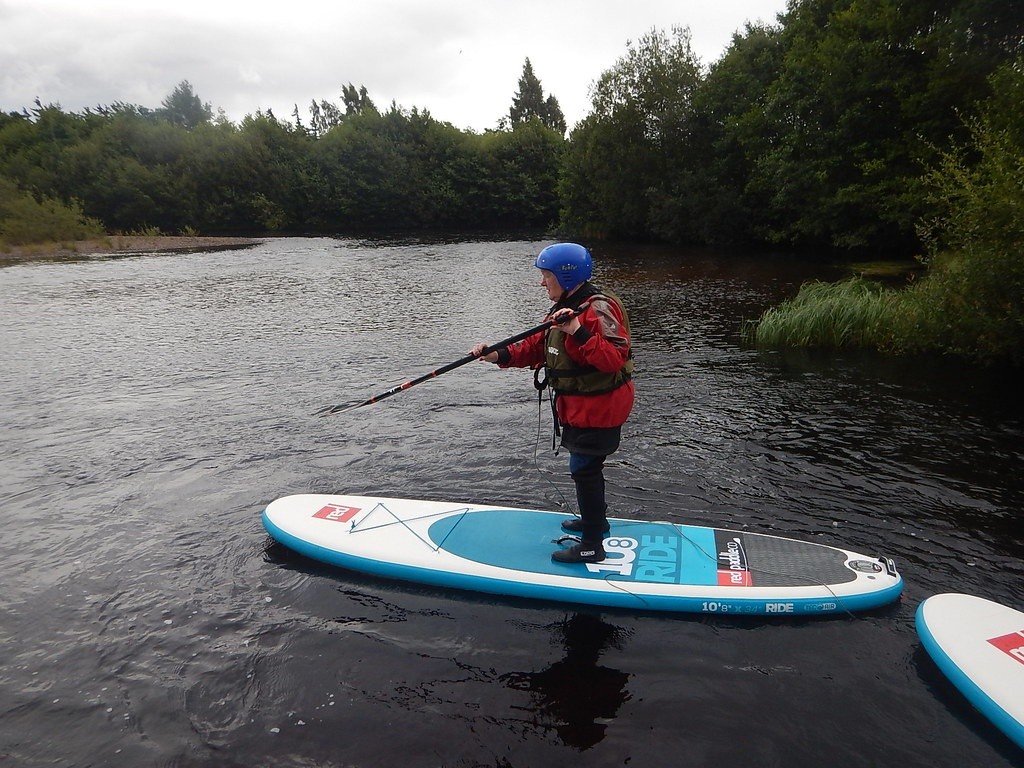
[535,242,592,291]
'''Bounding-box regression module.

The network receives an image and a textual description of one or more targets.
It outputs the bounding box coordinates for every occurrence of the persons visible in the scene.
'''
[472,243,636,564]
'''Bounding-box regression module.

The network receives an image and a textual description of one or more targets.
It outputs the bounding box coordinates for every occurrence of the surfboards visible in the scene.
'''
[914,591,1024,752]
[261,491,903,616]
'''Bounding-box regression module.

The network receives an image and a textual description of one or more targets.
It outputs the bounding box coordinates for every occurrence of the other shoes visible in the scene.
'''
[552,541,606,563]
[561,517,610,533]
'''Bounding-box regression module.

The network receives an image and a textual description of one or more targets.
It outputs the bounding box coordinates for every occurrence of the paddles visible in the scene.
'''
[312,302,589,416]
